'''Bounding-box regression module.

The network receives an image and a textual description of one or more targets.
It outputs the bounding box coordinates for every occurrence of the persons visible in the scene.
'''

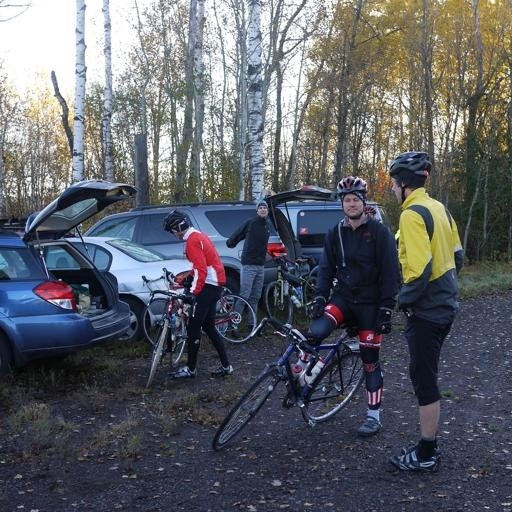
[387,149,464,471]
[283,177,398,438]
[364,208,376,217]
[227,198,270,340]
[163,209,235,379]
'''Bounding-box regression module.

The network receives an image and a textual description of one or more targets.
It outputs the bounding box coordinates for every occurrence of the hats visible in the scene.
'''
[256,201,268,212]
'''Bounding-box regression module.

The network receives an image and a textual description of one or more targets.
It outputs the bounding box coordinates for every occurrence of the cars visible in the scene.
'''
[34,237,193,344]
[0,178,143,379]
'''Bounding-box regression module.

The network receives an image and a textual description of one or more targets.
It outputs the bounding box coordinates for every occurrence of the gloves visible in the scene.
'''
[183,293,196,305]
[376,308,391,334]
[309,296,326,319]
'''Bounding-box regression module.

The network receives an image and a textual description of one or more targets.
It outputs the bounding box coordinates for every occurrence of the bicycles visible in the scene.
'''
[146,288,197,391]
[212,317,366,452]
[141,269,257,351]
[263,254,318,327]
[142,268,236,330]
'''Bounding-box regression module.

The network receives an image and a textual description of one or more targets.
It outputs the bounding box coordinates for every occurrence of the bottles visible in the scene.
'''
[296,286,303,300]
[290,351,328,391]
[289,293,302,309]
[169,311,178,333]
[283,279,289,296]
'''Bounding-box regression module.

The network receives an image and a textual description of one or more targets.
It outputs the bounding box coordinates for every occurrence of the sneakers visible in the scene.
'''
[249,326,263,337]
[172,365,196,378]
[211,365,234,378]
[390,451,438,473]
[358,416,381,436]
[401,445,441,463]
[231,326,239,338]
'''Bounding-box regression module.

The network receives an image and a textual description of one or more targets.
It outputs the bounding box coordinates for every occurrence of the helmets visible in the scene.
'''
[389,152,432,177]
[337,176,368,195]
[162,210,188,232]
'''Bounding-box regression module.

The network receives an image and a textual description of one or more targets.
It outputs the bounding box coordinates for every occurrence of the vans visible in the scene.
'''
[82,185,333,296]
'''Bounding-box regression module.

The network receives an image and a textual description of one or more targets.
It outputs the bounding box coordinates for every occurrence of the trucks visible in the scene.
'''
[275,199,392,288]
